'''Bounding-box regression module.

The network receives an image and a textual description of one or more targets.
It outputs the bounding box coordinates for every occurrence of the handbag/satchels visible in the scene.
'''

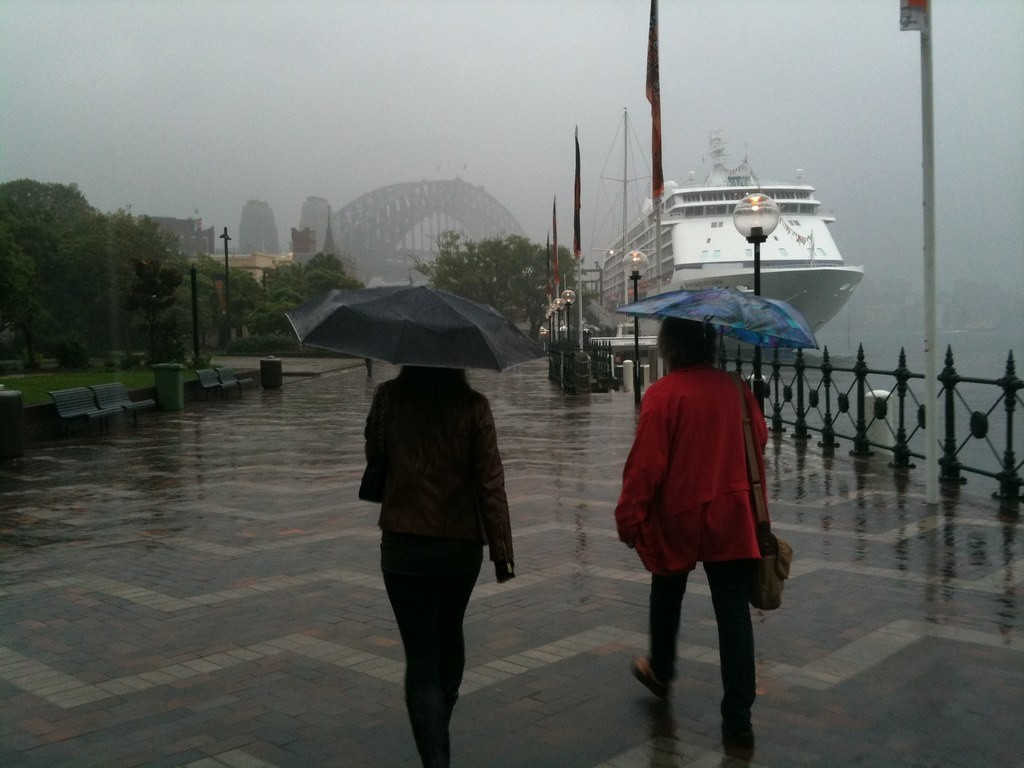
[359,381,386,504]
[747,533,793,610]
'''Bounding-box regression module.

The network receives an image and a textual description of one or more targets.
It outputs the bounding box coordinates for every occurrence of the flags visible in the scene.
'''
[642,0,663,200]
[546,232,553,294]
[573,136,582,256]
[552,200,560,288]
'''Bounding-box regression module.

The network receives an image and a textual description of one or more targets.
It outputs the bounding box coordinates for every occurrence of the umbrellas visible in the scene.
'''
[286,283,547,373]
[615,282,820,372]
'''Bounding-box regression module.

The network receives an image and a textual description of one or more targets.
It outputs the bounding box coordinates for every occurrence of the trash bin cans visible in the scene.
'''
[259,358,285,388]
[0,389,26,458]
[150,363,188,411]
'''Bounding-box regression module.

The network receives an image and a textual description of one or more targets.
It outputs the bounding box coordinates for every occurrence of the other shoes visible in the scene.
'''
[631,655,672,699]
[724,743,754,760]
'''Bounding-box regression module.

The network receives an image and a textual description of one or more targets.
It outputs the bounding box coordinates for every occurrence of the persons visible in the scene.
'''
[365,357,372,377]
[613,316,773,764]
[359,364,516,768]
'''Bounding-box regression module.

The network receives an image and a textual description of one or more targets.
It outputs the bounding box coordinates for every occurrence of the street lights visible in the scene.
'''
[732,192,782,420]
[622,251,650,404]
[544,290,576,345]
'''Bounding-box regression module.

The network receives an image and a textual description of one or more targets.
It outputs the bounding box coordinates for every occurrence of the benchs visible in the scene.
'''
[44,387,125,439]
[216,366,254,396]
[194,369,238,401]
[87,382,159,428]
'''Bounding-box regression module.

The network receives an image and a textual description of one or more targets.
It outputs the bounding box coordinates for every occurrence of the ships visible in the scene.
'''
[587,107,867,352]
